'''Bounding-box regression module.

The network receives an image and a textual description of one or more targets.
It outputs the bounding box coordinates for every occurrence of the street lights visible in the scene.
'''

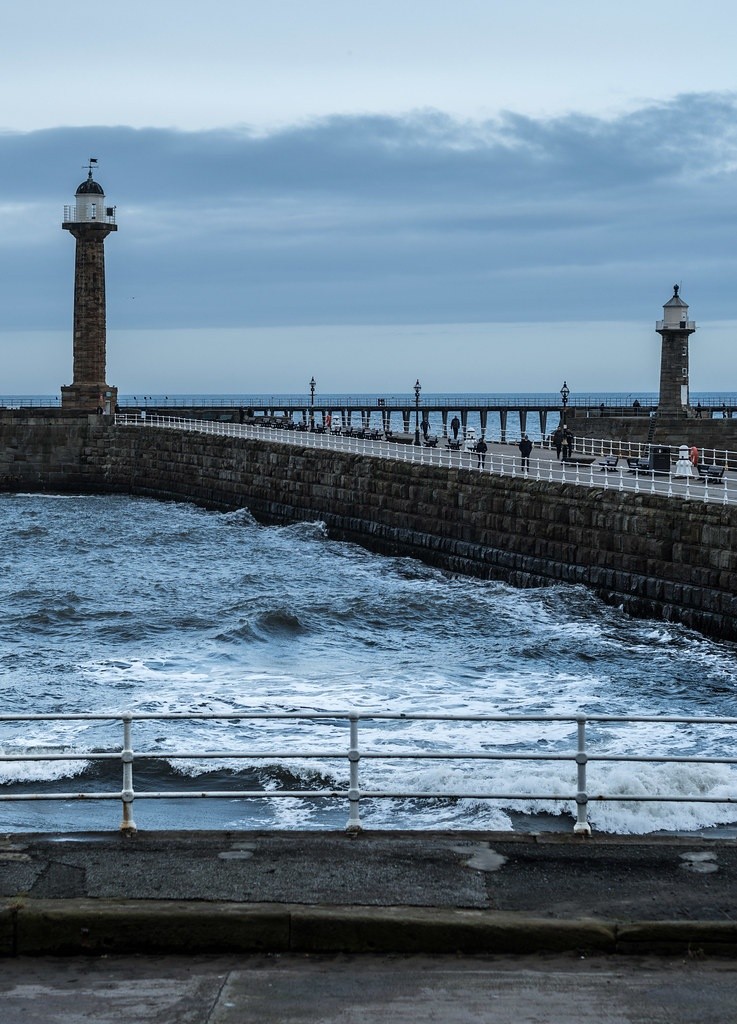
[411,378,423,447]
[560,380,570,464]
[307,376,318,433]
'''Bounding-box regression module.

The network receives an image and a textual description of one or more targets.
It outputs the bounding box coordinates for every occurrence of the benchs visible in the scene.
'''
[700,465,725,484]
[599,456,619,472]
[629,458,650,475]
[245,417,463,451]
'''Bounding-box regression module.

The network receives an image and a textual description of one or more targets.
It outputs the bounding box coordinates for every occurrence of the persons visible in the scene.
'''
[239,406,244,425]
[450,416,460,440]
[632,400,641,416]
[114,404,120,423]
[696,402,702,418]
[552,426,574,460]
[518,434,532,472]
[722,403,727,418]
[247,406,255,425]
[476,438,487,469]
[96,405,103,414]
[688,441,698,468]
[419,417,431,438]
[600,402,604,417]
[325,414,331,434]
